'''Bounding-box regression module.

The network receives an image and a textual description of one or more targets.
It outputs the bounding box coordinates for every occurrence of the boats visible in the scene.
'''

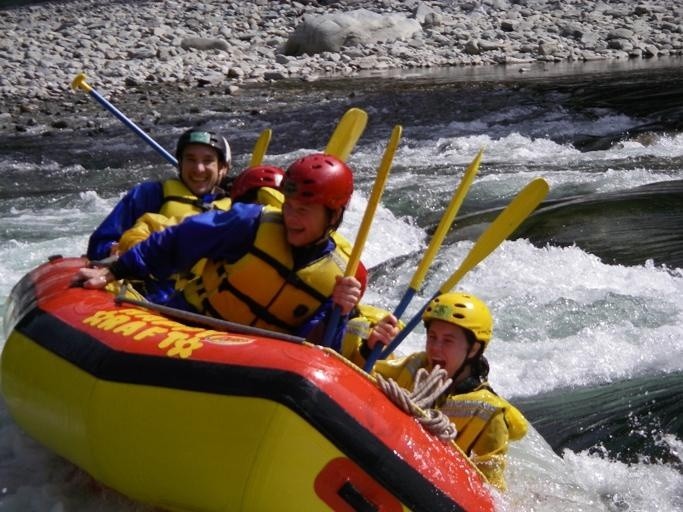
[1,249,505,512]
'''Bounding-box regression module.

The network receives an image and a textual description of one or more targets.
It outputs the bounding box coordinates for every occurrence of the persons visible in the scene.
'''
[74,151,367,358]
[345,291,509,494]
[116,165,288,295]
[87,125,230,303]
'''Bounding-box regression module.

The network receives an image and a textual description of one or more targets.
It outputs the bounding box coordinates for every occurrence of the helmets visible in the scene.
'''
[421,293,493,347]
[280,155,353,209]
[231,166,284,205]
[176,128,232,165]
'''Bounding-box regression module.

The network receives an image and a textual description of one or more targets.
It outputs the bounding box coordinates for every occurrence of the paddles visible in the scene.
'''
[321,105,370,164]
[72,74,182,170]
[325,123,403,350]
[375,177,549,362]
[249,128,273,167]
[360,148,487,374]
